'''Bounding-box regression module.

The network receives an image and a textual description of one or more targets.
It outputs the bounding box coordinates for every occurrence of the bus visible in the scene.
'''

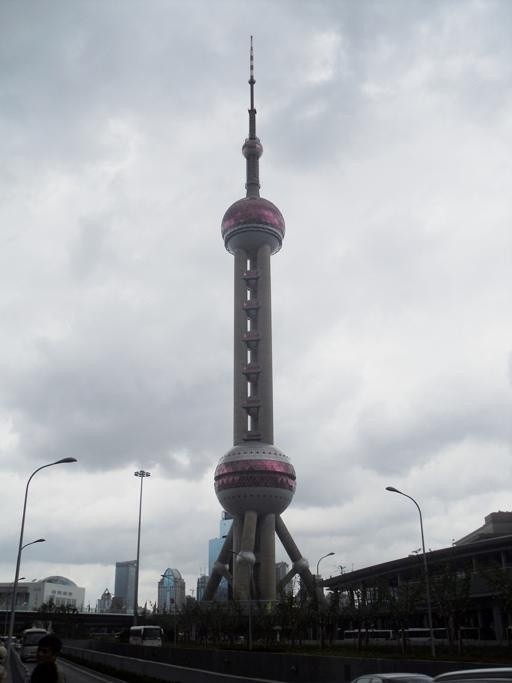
[274,626,496,647]
[128,625,165,650]
[21,626,51,662]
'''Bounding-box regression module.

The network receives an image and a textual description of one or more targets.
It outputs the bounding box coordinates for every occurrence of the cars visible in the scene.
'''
[342,667,512,682]
[0,635,22,648]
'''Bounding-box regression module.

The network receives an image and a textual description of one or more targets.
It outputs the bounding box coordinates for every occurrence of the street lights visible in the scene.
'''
[317,552,335,575]
[133,469,151,616]
[385,486,437,660]
[7,457,77,663]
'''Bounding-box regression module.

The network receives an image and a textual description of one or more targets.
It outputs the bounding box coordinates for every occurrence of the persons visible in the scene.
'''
[0,641,8,679]
[30,634,68,683]
